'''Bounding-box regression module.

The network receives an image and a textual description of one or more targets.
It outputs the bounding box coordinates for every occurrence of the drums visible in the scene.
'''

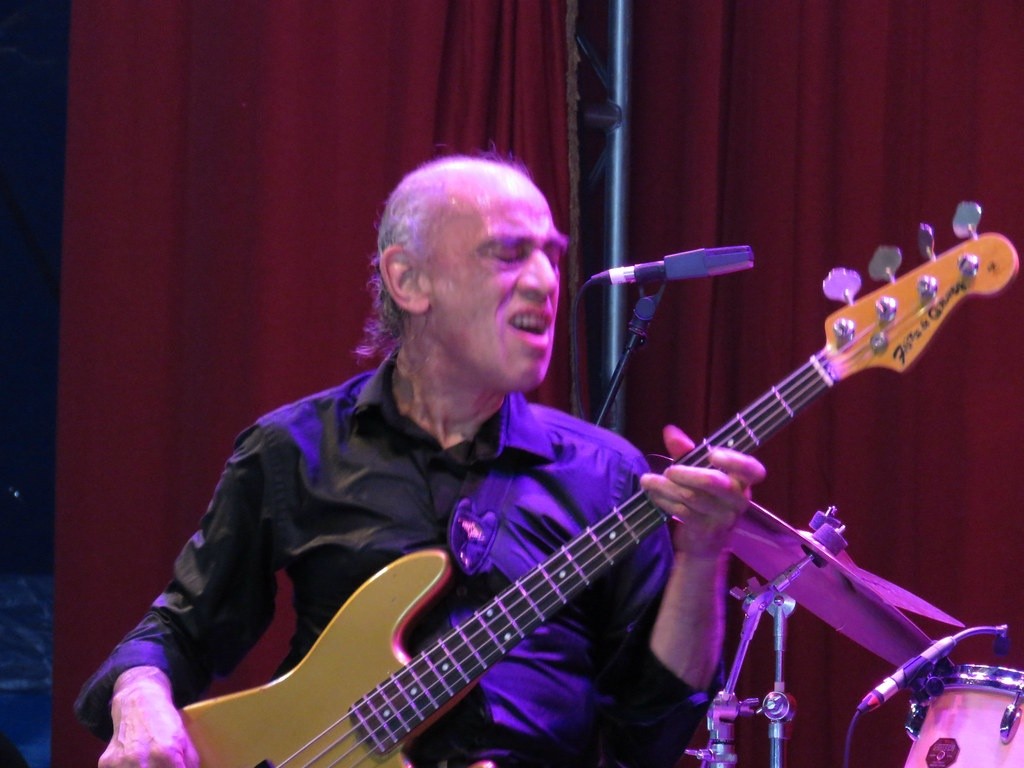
[904,662,1024,768]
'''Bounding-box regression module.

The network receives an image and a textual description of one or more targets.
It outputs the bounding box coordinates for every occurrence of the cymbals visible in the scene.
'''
[797,529,966,628]
[641,451,955,671]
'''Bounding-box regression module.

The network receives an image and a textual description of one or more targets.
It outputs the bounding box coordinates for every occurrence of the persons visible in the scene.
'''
[69,156,767,768]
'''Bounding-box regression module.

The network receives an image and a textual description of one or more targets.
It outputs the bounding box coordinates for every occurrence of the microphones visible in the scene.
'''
[591,245,754,284]
[856,625,1010,713]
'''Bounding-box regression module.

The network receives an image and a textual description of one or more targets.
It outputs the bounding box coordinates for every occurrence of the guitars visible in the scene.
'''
[168,196,1019,767]
